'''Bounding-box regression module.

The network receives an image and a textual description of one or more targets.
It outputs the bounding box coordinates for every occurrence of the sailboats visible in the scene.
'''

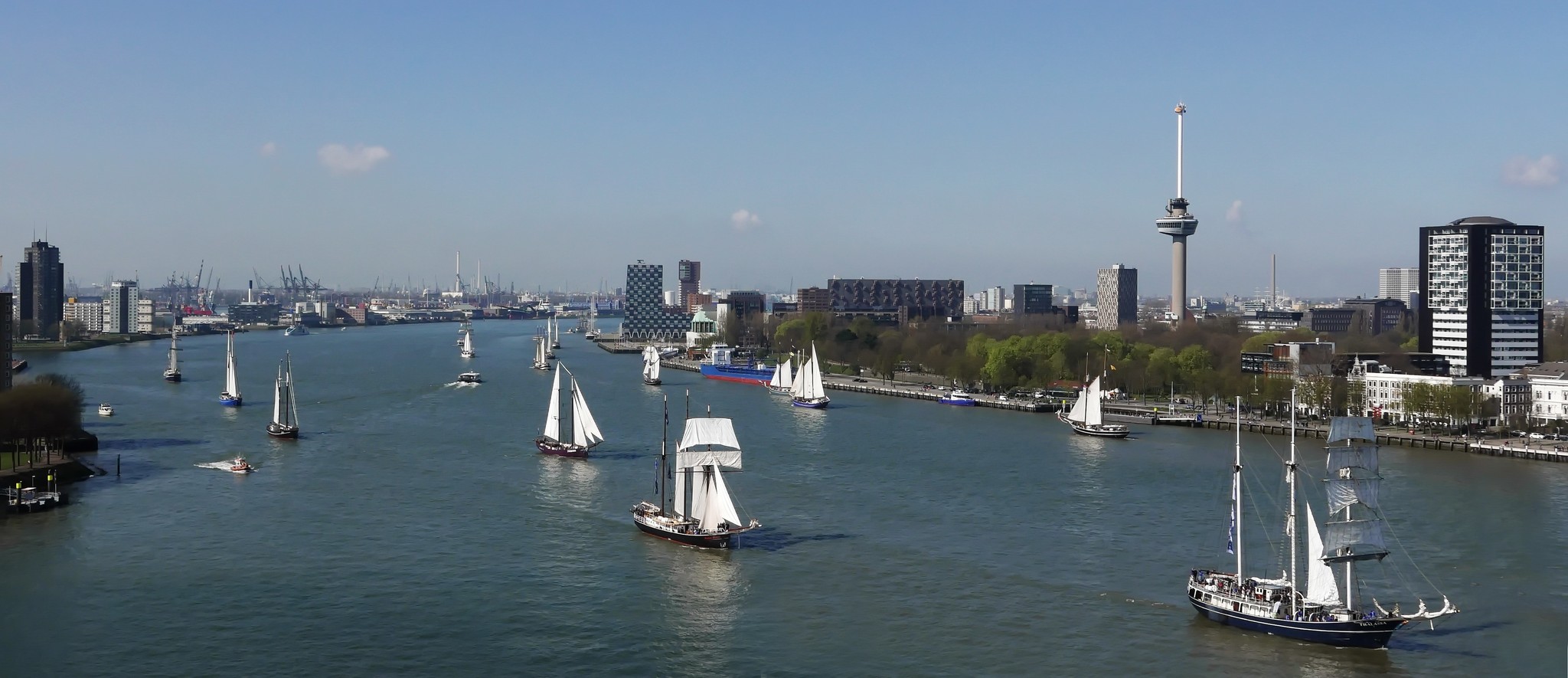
[1185,387,1461,646]
[163,312,184,382]
[629,387,763,549]
[162,260,557,316]
[219,328,242,407]
[266,349,299,438]
[642,339,662,385]
[532,359,605,459]
[1055,345,1130,439]
[768,338,831,408]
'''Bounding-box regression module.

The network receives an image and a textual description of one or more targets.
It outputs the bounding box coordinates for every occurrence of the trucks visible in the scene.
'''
[985,389,1079,401]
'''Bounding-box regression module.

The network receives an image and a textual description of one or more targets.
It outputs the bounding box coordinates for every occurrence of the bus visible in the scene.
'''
[905,367,911,372]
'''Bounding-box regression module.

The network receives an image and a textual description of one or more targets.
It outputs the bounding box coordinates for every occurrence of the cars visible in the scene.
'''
[1512,430,1527,435]
[1546,433,1560,438]
[938,386,946,391]
[1528,432,1545,439]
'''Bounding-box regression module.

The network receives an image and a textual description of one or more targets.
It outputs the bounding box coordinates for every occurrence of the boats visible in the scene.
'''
[284,323,310,336]
[533,313,562,371]
[938,389,976,406]
[22,335,52,342]
[457,370,482,383]
[566,294,601,340]
[98,402,116,415]
[230,456,250,474]
[341,327,347,331]
[452,319,476,358]
